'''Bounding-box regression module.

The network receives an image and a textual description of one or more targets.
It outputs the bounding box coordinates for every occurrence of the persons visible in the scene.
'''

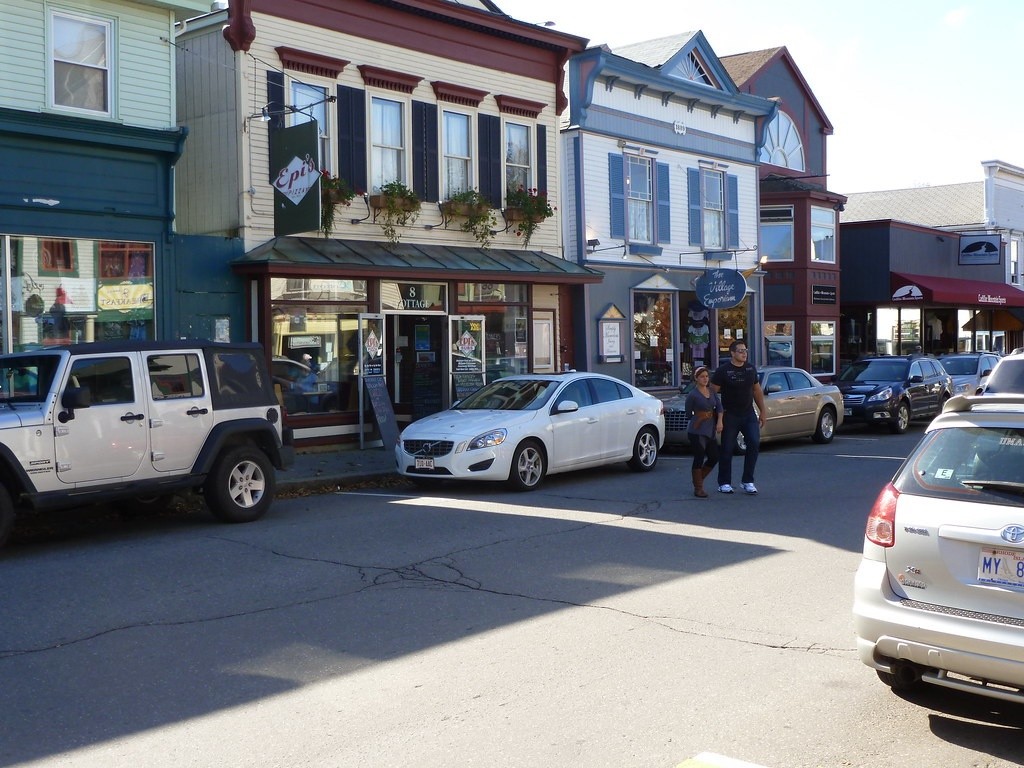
[685,366,722,497]
[710,341,766,494]
[298,360,319,407]
[916,345,922,353]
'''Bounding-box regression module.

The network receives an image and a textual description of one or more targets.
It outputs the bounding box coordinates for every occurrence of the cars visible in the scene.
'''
[853,392,1024,712]
[395,371,665,492]
[660,365,845,456]
[271,354,316,390]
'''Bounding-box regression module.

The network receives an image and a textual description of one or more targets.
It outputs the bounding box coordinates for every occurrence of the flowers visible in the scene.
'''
[503,183,557,248]
[375,180,421,242]
[439,186,497,232]
[320,170,364,200]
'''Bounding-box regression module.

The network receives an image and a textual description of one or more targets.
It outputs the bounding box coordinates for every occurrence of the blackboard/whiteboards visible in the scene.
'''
[364,376,401,450]
[452,373,485,402]
[411,362,443,421]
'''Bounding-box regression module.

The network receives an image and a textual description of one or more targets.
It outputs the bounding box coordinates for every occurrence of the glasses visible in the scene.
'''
[733,349,749,353]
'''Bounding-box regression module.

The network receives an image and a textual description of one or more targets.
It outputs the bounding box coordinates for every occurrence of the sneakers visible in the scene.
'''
[718,484,734,493]
[740,482,758,493]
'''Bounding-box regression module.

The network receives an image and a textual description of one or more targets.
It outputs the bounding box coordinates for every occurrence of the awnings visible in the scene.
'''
[891,272,1024,309]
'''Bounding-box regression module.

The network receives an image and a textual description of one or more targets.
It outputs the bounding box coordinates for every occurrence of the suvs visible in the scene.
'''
[0,340,283,551]
[940,351,1004,397]
[981,347,1024,399]
[835,354,954,435]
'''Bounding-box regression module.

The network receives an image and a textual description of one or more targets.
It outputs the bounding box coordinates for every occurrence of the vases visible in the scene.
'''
[369,196,420,213]
[443,201,487,216]
[322,189,341,204]
[504,208,544,223]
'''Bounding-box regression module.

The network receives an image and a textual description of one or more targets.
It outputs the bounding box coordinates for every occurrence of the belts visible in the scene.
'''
[694,410,713,430]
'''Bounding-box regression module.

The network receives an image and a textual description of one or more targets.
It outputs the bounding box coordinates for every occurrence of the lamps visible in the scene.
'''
[490,208,508,235]
[351,192,370,223]
[587,242,628,259]
[638,255,669,273]
[425,201,444,230]
[261,100,296,122]
[729,245,758,253]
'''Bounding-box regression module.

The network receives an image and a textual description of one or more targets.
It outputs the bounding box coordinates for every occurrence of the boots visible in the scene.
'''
[692,468,708,497]
[693,464,711,485]
